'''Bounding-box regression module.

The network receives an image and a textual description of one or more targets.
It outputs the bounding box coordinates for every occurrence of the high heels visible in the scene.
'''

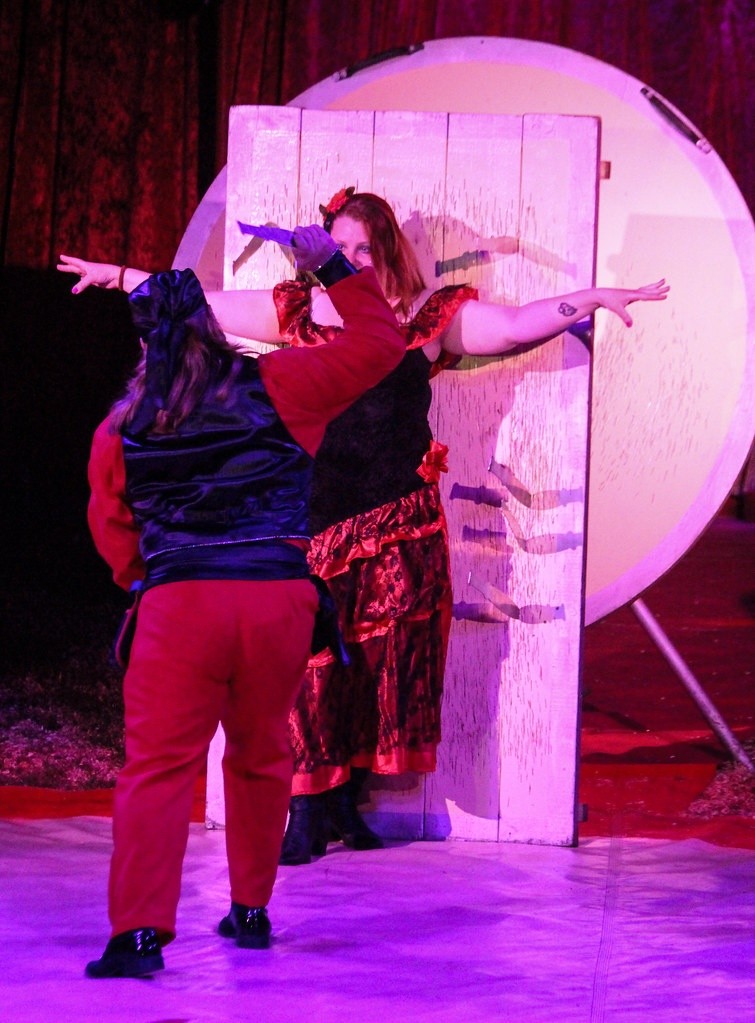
[278,793,329,865]
[326,767,384,850]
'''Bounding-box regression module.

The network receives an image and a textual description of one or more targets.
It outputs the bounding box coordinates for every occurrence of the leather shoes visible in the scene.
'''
[86,928,164,978]
[218,902,271,949]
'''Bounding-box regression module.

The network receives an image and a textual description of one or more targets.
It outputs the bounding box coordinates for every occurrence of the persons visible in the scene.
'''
[57,186,670,865]
[85,224,406,978]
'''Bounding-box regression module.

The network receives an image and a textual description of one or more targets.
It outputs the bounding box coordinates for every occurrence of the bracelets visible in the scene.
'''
[118,265,127,291]
